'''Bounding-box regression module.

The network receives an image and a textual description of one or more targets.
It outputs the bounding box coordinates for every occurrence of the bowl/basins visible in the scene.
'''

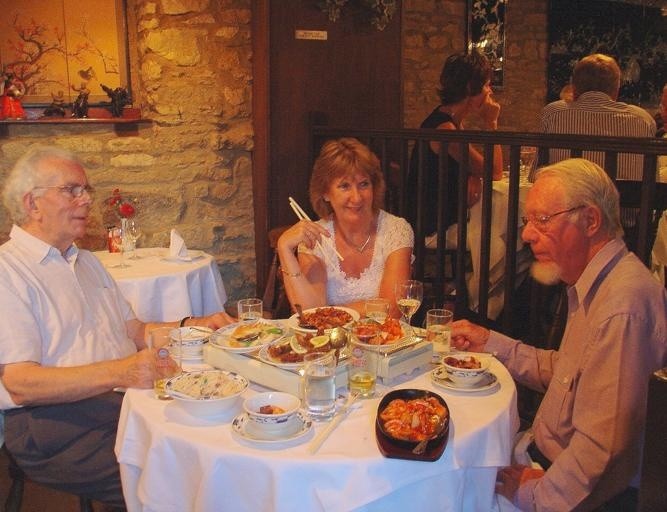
[243,391,301,430]
[170,326,212,345]
[163,369,249,414]
[442,355,491,384]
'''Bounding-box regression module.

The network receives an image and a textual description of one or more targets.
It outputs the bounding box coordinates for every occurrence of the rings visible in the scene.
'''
[305,230,311,237]
[475,192,479,197]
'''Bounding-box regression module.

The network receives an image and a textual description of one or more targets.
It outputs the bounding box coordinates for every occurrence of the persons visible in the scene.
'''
[408,49,504,250]
[277,136,415,321]
[0,142,237,511]
[446,158,667,512]
[529,52,656,235]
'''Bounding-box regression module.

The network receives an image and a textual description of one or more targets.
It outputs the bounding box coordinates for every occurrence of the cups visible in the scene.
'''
[146,327,181,399]
[364,297,389,323]
[237,297,263,319]
[427,309,453,363]
[348,326,380,395]
[303,351,337,421]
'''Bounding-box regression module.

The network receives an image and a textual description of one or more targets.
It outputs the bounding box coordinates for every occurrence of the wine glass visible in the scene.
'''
[111,219,142,268]
[395,279,424,324]
[518,144,537,186]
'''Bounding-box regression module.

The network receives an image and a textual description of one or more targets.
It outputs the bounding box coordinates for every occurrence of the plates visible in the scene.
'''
[258,333,300,369]
[350,317,414,350]
[232,412,313,443]
[430,366,499,392]
[289,307,359,332]
[375,388,450,462]
[175,354,203,360]
[208,319,289,354]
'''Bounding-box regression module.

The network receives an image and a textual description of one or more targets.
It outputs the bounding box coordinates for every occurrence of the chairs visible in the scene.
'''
[637,367,667,512]
[414,242,473,318]
[225,222,296,321]
[527,177,667,350]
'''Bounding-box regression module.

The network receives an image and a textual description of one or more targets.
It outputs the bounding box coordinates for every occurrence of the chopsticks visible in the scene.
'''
[288,196,345,273]
[307,392,359,455]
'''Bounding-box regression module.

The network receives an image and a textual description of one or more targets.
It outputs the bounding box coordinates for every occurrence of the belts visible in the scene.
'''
[527,441,552,471]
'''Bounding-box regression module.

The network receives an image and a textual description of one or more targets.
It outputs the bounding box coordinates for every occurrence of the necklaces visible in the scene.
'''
[443,106,460,119]
[339,225,373,254]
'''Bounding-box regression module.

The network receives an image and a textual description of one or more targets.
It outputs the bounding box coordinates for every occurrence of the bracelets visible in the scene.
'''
[279,265,302,279]
[180,316,194,327]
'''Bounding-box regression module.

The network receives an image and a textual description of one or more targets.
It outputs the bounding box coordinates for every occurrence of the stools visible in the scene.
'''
[1,440,94,512]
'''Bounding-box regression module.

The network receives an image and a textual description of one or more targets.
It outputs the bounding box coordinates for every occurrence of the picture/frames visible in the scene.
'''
[0,1,132,108]
[465,0,507,92]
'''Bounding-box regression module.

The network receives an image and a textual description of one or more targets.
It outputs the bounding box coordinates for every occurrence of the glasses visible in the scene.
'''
[521,205,586,231]
[33,183,95,198]
